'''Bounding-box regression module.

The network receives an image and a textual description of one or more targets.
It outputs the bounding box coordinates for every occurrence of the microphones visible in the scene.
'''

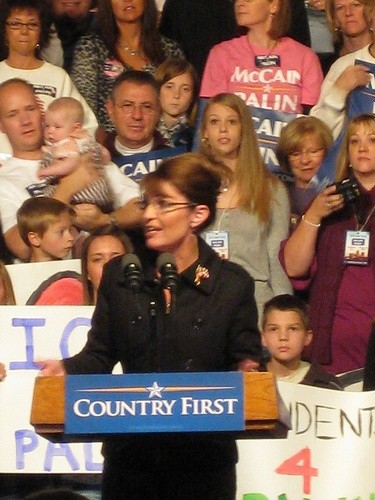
[155,254,177,290]
[121,254,146,289]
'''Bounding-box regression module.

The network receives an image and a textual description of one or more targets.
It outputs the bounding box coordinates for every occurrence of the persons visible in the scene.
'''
[201,0,325,113]
[20,197,76,262]
[273,115,334,229]
[201,91,293,339]
[0,0,98,140]
[309,0,375,143]
[153,57,198,147]
[326,0,372,59]
[159,0,342,78]
[277,114,375,397]
[81,224,134,305]
[0,78,147,266]
[258,292,344,391]
[0,262,16,305]
[71,0,185,137]
[38,152,269,500]
[104,70,170,160]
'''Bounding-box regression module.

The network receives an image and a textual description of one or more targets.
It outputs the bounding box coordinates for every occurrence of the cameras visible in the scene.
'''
[326,178,361,204]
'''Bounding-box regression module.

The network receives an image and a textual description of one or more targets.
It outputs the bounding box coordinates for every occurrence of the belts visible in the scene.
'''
[338,367,375,388]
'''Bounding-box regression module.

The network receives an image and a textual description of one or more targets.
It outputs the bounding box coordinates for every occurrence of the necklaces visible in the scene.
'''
[117,36,140,56]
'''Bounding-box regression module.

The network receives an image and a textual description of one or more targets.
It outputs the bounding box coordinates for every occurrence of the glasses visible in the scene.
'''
[111,97,162,116]
[3,18,43,31]
[288,146,328,161]
[136,196,206,212]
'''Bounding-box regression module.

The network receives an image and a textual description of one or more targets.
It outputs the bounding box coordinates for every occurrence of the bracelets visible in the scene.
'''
[109,212,118,228]
[302,214,321,228]
